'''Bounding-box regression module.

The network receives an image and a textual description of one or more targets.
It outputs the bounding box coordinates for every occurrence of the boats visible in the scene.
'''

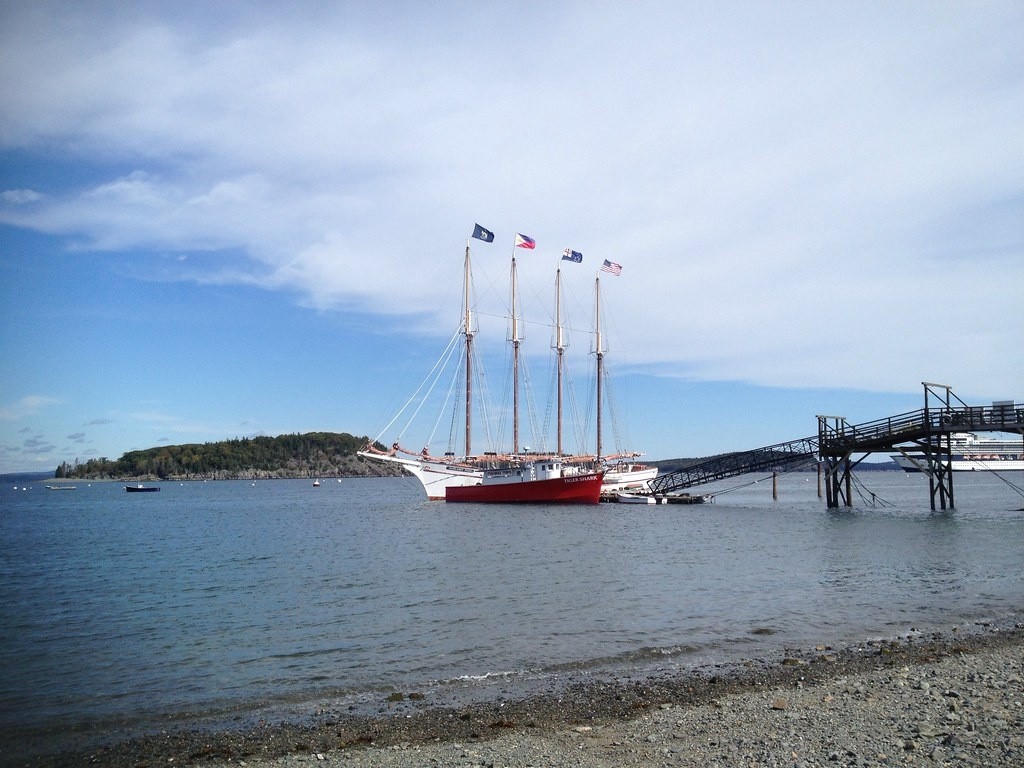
[353,222,660,501]
[444,446,606,505]
[616,491,666,505]
[126,484,160,492]
[665,493,703,504]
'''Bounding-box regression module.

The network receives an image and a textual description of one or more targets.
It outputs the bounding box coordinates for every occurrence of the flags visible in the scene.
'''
[515,232,536,250]
[560,248,582,263]
[471,224,494,244]
[600,259,622,276]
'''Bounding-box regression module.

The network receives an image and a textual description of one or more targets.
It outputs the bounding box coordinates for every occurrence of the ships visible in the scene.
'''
[890,431,1024,472]
